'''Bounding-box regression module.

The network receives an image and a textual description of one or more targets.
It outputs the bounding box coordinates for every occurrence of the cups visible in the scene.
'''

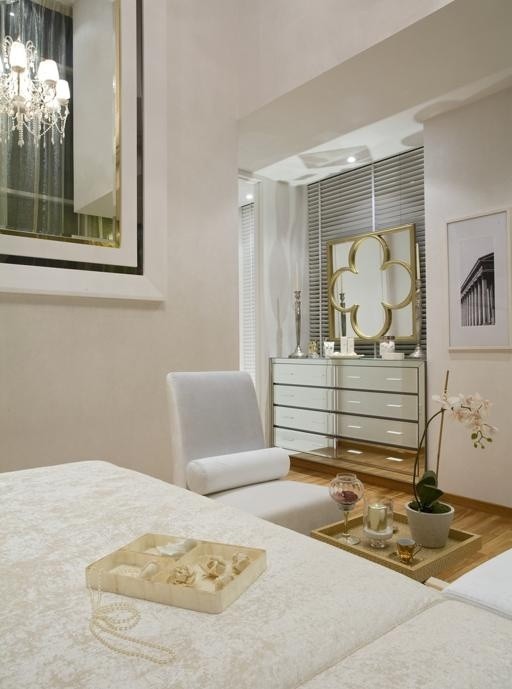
[361,495,395,548]
[324,341,335,357]
[395,538,422,564]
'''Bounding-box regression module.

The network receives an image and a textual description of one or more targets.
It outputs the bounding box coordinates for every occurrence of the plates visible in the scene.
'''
[327,352,366,360]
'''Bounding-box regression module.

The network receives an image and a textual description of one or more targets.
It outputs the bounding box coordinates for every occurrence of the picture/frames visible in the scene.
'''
[442,207,512,351]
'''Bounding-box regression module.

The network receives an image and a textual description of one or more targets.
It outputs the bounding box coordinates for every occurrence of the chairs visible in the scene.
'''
[164,369,345,536]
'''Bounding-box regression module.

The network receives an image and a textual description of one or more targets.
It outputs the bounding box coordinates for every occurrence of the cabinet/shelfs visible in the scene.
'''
[270,358,426,459]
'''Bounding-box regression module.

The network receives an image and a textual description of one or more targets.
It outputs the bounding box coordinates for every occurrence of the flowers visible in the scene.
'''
[409,392,500,512]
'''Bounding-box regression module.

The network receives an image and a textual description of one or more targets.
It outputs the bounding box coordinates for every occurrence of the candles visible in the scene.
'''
[415,243,421,280]
[367,505,387,532]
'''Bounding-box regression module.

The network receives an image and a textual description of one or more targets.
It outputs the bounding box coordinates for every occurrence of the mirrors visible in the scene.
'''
[326,222,419,345]
[0,1,167,307]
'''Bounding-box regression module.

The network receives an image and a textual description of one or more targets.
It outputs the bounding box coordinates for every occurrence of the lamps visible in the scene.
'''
[0,2,70,148]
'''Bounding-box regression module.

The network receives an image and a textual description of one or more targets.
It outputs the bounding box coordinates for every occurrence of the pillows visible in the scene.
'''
[186,446,291,496]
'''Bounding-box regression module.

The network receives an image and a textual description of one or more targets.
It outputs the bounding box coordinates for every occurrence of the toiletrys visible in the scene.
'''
[340,336,347,353]
[347,336,354,353]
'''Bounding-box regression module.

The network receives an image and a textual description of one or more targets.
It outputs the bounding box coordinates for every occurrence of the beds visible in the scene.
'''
[0,460,510,689]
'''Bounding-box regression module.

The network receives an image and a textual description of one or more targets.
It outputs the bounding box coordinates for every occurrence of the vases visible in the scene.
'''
[406,500,454,550]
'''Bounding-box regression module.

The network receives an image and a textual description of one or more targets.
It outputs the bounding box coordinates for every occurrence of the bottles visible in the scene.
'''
[380,335,395,358]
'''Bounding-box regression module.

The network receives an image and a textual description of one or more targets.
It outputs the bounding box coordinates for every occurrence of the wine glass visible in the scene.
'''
[326,474,364,547]
